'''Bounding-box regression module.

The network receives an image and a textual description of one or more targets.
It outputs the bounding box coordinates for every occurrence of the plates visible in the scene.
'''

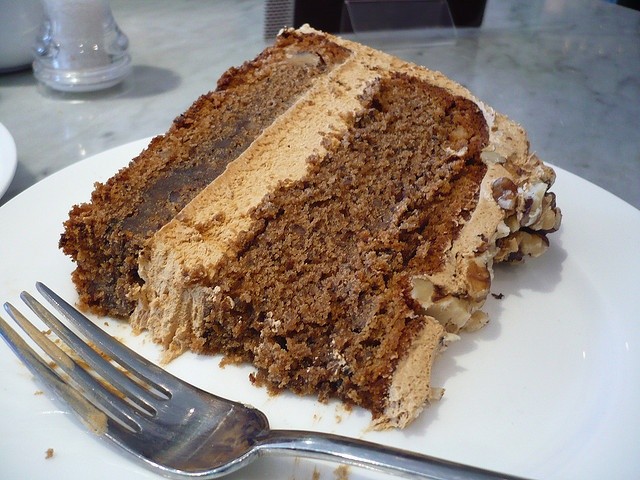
[0,122,18,200]
[0,133,640,480]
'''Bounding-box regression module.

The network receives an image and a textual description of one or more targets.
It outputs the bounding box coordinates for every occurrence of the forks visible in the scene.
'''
[0,283,532,480]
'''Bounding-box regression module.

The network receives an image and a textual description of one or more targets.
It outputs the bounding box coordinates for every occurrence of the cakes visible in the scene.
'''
[59,23,562,433]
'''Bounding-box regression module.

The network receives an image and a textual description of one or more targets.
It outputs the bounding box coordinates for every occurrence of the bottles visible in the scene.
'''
[33,0,131,94]
